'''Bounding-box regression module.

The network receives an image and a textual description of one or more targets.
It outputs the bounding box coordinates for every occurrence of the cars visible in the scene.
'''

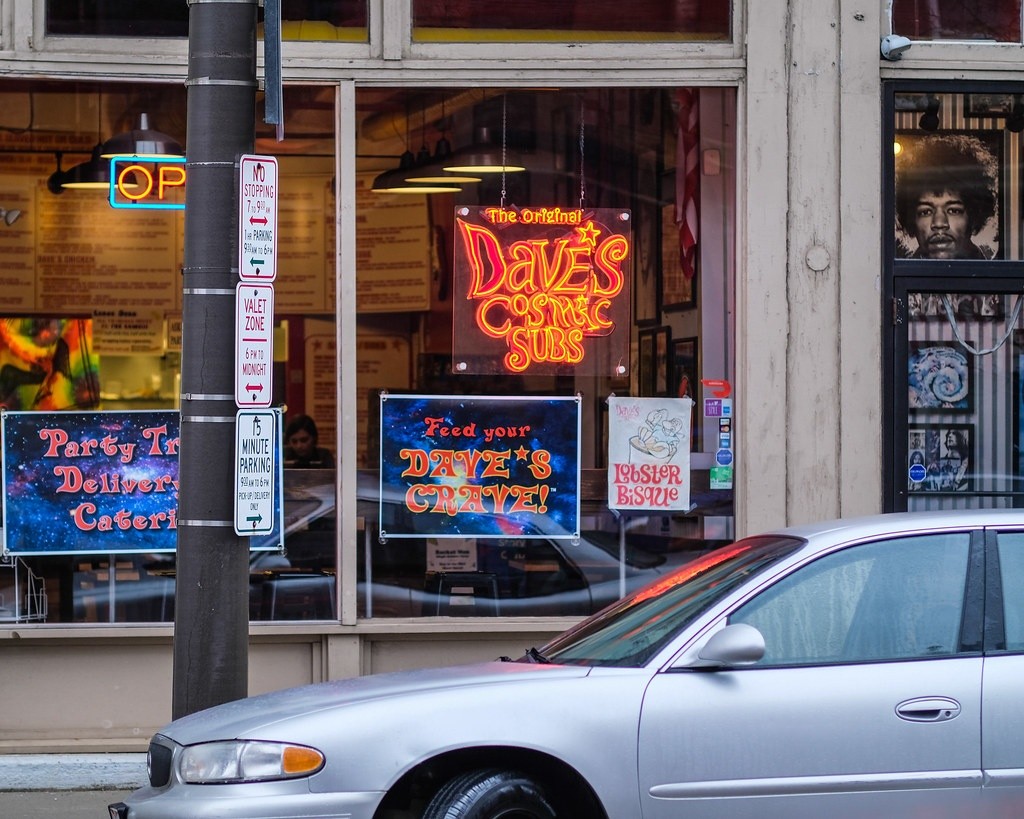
[73,479,709,622]
[106,508,1024,819]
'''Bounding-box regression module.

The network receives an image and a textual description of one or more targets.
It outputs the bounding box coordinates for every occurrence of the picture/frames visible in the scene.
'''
[907,422,976,494]
[894,93,936,113]
[908,339,975,415]
[962,94,1016,119]
[894,126,1012,324]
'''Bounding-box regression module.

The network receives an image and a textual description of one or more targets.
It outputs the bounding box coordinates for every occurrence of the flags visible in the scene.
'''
[665,87,702,282]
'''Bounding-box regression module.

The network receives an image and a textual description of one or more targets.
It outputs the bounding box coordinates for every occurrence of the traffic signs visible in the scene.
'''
[235,282,274,409]
[239,154,278,283]
[235,407,277,538]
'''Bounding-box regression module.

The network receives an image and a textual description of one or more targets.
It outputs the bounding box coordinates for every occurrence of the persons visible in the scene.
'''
[907,428,969,492]
[282,414,336,470]
[894,138,998,261]
[905,292,1000,319]
